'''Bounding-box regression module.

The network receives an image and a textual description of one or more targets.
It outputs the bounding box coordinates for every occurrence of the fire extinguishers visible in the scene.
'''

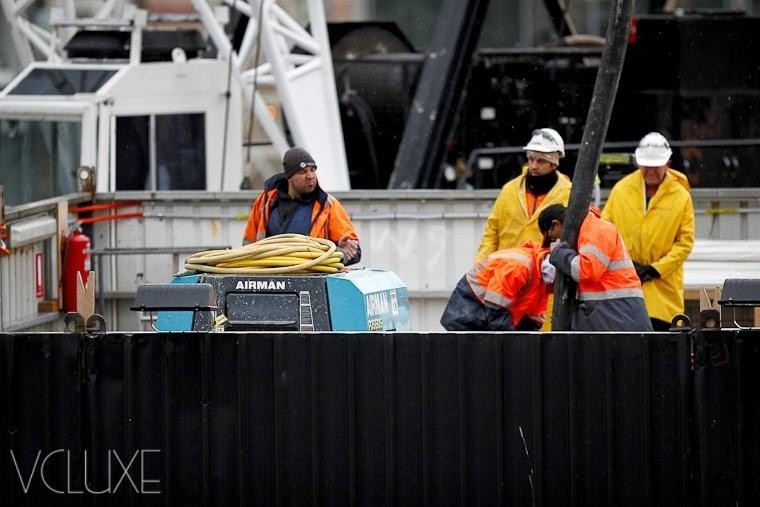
[59,220,92,313]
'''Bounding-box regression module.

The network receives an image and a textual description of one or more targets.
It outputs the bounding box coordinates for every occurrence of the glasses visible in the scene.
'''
[533,130,560,145]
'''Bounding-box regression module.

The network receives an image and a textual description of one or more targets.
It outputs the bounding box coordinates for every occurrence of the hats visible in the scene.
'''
[537,201,568,251]
[522,127,565,160]
[634,131,673,170]
[281,147,316,178]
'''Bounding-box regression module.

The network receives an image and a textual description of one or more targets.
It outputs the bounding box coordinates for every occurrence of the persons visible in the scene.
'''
[538,203,653,332]
[439,240,558,331]
[475,128,573,332]
[240,146,362,266]
[600,132,695,331]
